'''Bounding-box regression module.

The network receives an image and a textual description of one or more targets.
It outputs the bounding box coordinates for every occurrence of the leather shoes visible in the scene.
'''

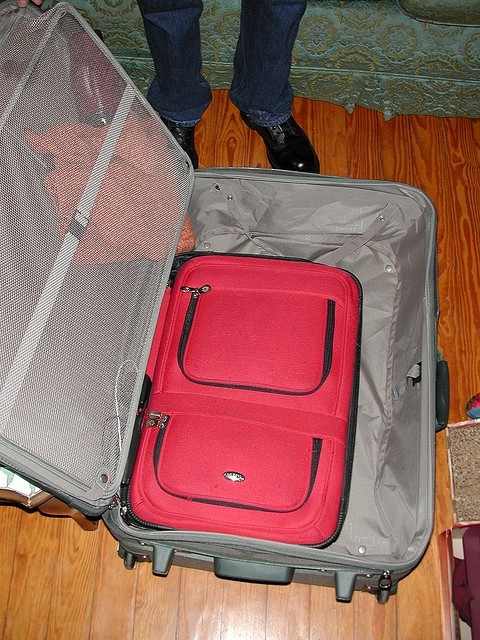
[160,116,199,168]
[239,112,319,173]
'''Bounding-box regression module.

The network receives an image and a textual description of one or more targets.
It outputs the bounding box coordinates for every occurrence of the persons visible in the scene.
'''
[16,1,323,172]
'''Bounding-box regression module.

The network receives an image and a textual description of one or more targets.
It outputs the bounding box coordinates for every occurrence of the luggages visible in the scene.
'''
[0,2,450,604]
[121,251,363,549]
